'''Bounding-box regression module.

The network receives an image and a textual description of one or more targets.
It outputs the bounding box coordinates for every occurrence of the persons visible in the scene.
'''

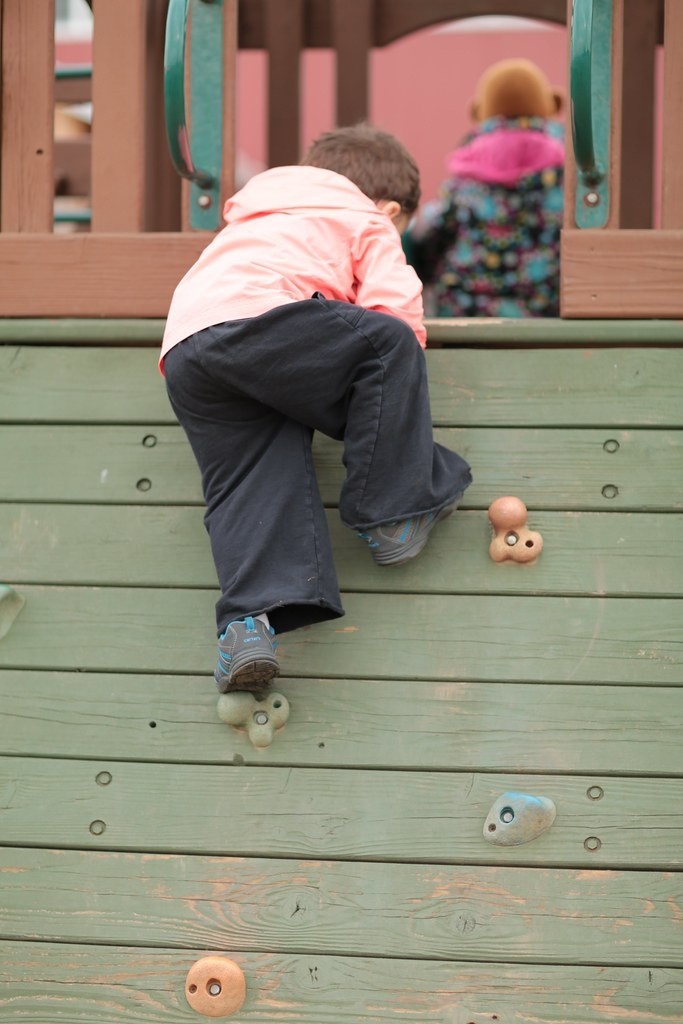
[159,125,472,694]
[404,57,566,320]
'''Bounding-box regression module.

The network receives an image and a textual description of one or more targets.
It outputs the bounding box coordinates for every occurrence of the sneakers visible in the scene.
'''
[357,491,465,569]
[214,617,281,694]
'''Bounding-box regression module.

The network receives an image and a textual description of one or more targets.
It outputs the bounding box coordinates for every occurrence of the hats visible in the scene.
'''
[470,60,563,120]
